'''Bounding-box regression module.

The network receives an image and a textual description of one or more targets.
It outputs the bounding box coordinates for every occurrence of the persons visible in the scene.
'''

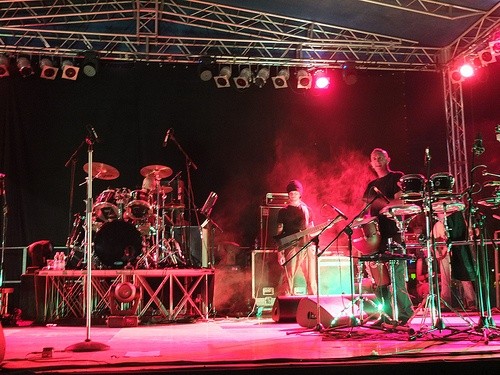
[430,212,476,312]
[276,180,317,297]
[417,248,440,296]
[362,148,413,327]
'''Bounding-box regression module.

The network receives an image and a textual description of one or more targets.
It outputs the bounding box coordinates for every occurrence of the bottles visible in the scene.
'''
[59,251,66,270]
[53,252,60,271]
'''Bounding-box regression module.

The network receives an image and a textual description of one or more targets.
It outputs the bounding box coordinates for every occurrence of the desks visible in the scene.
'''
[37,269,216,323]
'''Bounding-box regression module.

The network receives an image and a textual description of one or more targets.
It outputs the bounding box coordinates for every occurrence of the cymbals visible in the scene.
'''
[150,185,173,195]
[140,164,173,178]
[157,203,186,209]
[83,162,120,181]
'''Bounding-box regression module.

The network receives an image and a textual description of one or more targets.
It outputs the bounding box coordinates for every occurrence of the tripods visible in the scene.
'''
[134,170,187,269]
[285,149,500,343]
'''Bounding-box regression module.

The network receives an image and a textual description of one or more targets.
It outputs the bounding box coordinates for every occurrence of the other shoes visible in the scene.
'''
[372,317,391,326]
[399,316,410,325]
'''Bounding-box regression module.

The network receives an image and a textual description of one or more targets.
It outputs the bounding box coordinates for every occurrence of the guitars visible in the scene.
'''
[273,218,342,252]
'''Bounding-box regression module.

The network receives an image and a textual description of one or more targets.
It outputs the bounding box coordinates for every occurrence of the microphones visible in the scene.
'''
[476,135,482,156]
[425,148,430,163]
[329,203,348,220]
[92,128,98,138]
[373,186,390,204]
[164,129,170,142]
[458,185,475,199]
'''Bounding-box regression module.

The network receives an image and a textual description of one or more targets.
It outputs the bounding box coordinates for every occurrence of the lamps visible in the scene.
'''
[448,31,500,84]
[0,50,101,81]
[107,279,142,328]
[197,56,359,88]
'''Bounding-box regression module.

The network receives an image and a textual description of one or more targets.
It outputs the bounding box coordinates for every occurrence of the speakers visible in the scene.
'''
[206,265,256,316]
[272,293,381,328]
[317,257,354,295]
[169,225,208,268]
[258,205,285,250]
[29,239,53,269]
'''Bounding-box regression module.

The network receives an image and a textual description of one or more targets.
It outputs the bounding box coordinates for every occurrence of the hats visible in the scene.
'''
[286,179,303,196]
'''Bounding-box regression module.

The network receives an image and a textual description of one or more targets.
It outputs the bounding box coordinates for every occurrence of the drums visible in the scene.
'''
[349,216,391,286]
[401,211,449,261]
[64,188,163,269]
[389,172,466,216]
